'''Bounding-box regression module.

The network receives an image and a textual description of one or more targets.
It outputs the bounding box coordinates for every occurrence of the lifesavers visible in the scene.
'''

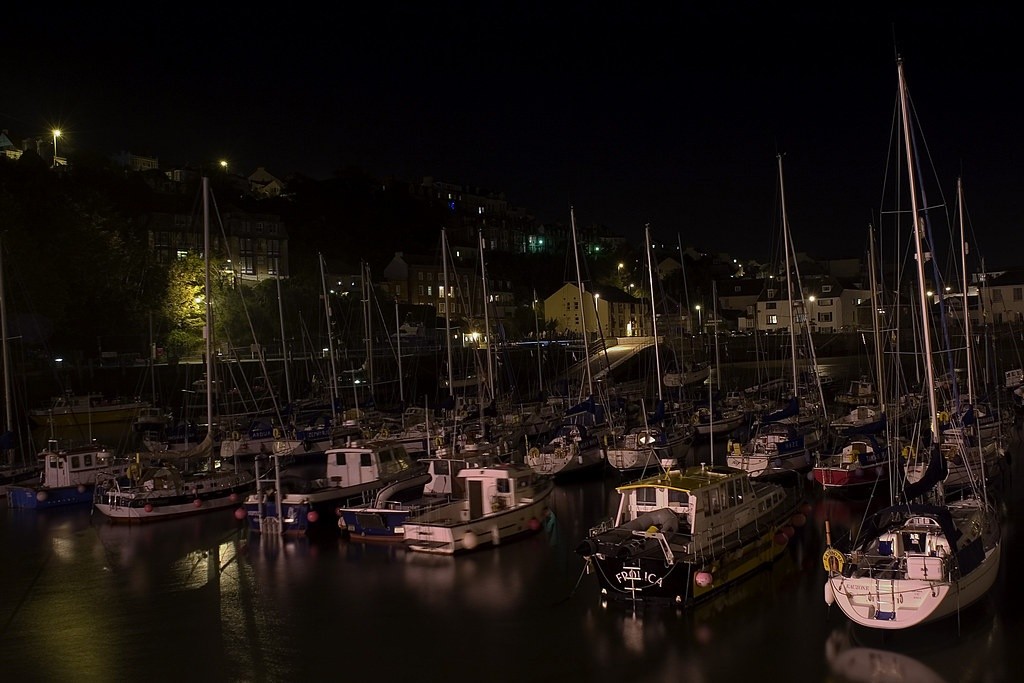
[127,464,142,479]
[850,450,860,461]
[529,448,540,458]
[940,411,949,422]
[638,432,646,446]
[380,429,389,438]
[822,548,847,573]
[273,428,279,439]
[554,448,565,458]
[903,446,915,460]
[436,438,442,446]
[232,431,239,439]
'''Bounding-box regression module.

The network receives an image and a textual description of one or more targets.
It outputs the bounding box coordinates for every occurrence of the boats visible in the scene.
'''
[8,446,138,511]
[834,374,886,407]
[28,393,147,428]
[401,463,555,555]
[334,455,482,541]
[235,440,428,540]
[576,457,816,616]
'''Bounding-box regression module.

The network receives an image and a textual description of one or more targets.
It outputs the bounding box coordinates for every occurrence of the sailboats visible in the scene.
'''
[6,150,1023,522]
[820,56,1004,631]
